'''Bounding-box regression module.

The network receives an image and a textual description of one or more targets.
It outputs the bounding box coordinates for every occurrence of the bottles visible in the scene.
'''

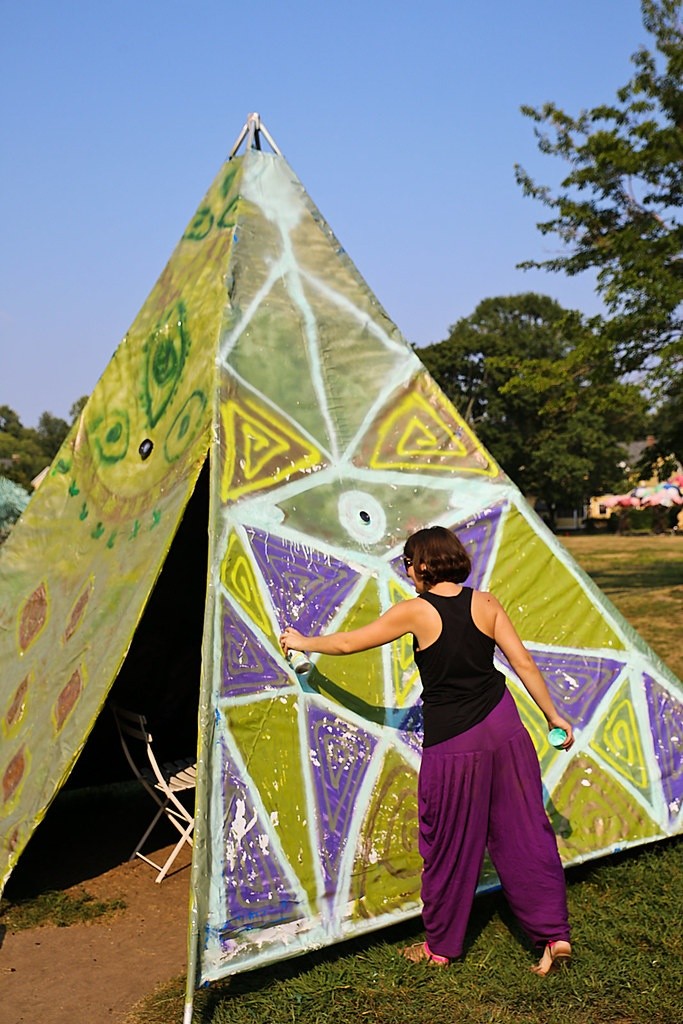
[285,631,312,676]
[548,728,567,750]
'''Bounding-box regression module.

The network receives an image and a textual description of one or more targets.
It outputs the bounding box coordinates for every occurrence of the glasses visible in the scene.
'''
[403,557,413,571]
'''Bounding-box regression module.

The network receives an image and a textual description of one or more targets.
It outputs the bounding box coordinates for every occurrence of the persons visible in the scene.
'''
[280,527,575,980]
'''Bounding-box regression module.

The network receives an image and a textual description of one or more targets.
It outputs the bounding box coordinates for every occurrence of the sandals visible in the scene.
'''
[397,941,448,968]
[530,941,572,977]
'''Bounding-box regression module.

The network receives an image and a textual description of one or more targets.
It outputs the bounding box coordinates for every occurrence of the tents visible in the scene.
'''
[0,109,683,1024]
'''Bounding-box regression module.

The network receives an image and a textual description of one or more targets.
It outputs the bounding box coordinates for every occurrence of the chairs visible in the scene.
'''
[111,703,198,884]
